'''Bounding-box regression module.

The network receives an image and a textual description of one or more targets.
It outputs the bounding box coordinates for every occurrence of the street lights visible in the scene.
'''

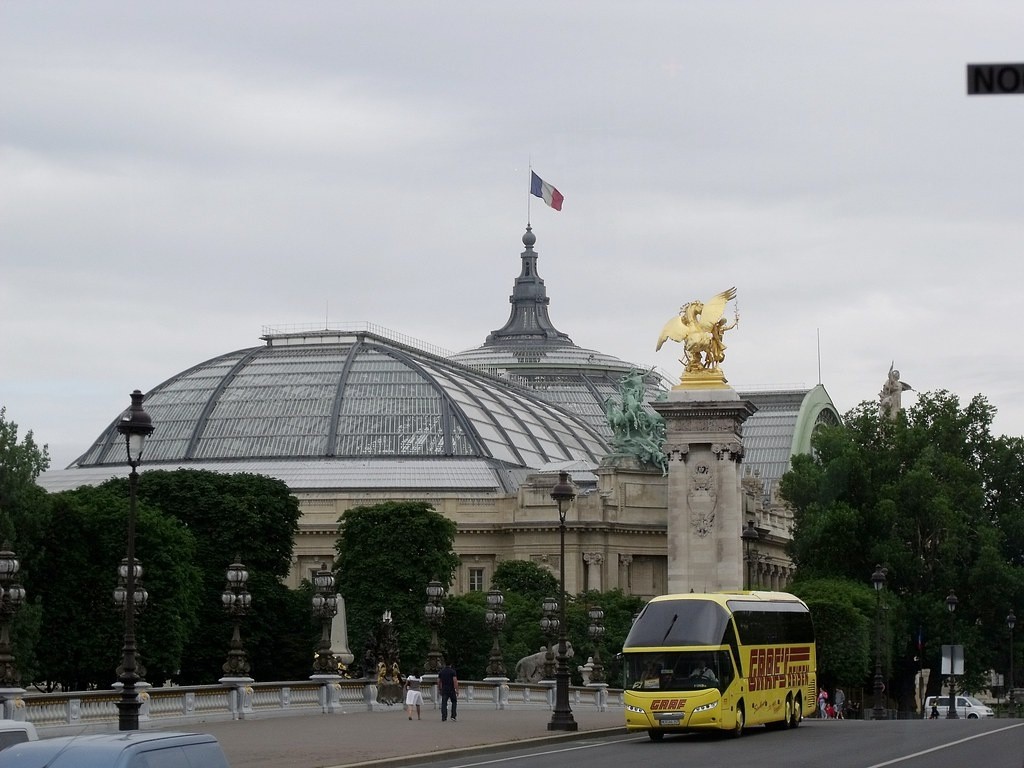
[550,469,581,733]
[1005,607,1017,719]
[944,587,962,719]
[115,390,160,731]
[869,562,888,720]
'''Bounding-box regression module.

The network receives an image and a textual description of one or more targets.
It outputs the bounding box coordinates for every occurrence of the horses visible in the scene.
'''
[679,299,725,365]
[603,386,646,437]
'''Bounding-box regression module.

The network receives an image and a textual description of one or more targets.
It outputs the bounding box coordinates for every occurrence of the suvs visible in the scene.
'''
[0,730,230,768]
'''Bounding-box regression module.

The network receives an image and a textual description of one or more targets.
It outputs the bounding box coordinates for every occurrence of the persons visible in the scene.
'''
[335,656,349,673]
[928,701,939,720]
[617,365,657,402]
[817,687,861,719]
[689,660,718,682]
[888,361,917,419]
[404,667,425,720]
[703,319,738,369]
[437,661,461,722]
[641,661,672,684]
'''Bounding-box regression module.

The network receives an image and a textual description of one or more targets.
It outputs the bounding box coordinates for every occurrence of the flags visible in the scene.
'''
[529,169,564,211]
[918,629,923,651]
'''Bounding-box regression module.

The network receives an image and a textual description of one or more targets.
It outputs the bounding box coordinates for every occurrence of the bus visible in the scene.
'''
[614,589,820,741]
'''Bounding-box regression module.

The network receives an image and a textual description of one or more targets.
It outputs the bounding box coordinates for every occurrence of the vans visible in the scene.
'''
[0,718,40,752]
[923,695,995,720]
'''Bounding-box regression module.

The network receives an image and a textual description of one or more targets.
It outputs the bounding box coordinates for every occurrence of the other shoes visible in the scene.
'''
[409,717,412,720]
[451,717,456,721]
[442,718,447,721]
